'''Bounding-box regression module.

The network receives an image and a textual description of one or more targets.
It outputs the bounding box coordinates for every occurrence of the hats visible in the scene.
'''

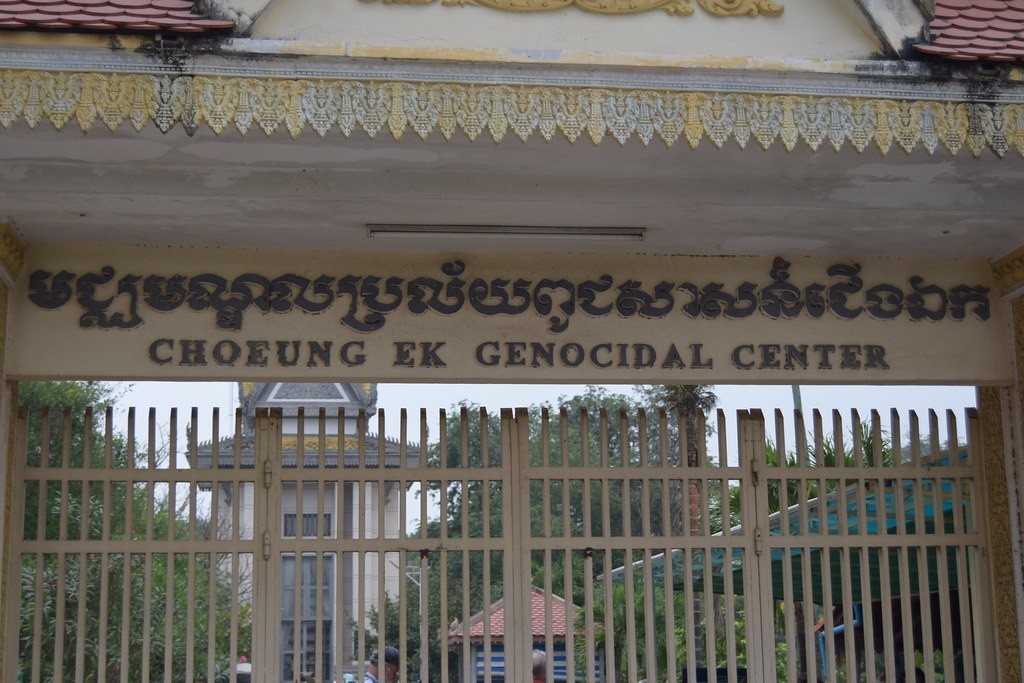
[372,646,412,670]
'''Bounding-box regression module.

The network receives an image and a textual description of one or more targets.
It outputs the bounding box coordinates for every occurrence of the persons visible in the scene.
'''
[363,646,399,683]
[532,648,547,683]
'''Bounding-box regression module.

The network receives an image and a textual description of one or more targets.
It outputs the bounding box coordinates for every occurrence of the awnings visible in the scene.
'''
[598,444,970,608]
